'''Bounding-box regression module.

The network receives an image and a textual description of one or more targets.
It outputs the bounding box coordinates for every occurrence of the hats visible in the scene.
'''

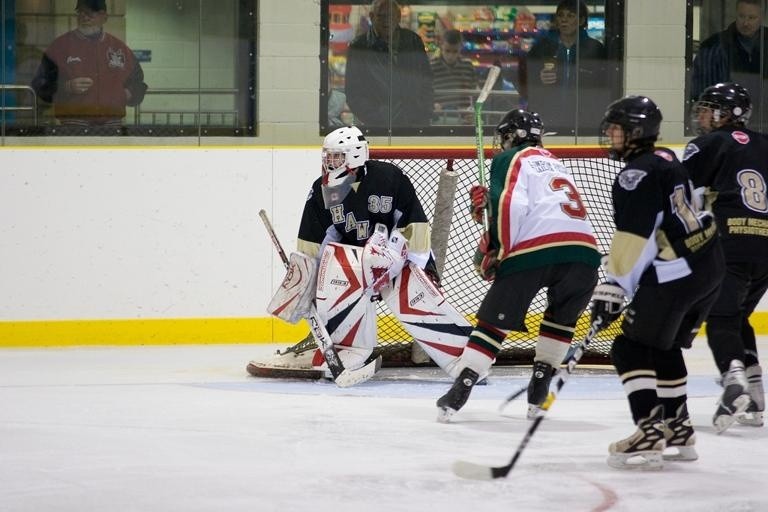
[76,0,106,12]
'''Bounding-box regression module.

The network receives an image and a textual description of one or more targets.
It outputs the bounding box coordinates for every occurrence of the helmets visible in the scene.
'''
[599,95,662,161]
[494,110,544,154]
[321,126,369,189]
[690,81,753,136]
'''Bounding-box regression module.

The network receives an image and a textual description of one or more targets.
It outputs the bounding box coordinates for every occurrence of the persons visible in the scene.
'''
[518,0,606,137]
[596,95,728,453]
[436,109,602,410]
[680,82,768,425]
[253,124,440,369]
[30,1,148,126]
[431,28,477,118]
[694,0,768,99]
[344,0,433,135]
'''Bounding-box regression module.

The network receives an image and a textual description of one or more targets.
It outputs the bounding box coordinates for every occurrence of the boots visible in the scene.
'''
[437,367,478,410]
[528,362,555,406]
[713,360,765,424]
[609,402,695,453]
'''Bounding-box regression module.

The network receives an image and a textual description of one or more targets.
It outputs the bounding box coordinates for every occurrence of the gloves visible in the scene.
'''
[591,279,626,330]
[470,185,492,224]
[473,231,499,280]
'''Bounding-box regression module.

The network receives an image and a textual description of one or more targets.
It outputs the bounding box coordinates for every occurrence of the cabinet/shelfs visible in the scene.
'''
[447,12,553,76]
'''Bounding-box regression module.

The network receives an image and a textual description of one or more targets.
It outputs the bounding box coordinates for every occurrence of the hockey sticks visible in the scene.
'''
[473,65,502,231]
[448,318,602,481]
[258,208,383,388]
